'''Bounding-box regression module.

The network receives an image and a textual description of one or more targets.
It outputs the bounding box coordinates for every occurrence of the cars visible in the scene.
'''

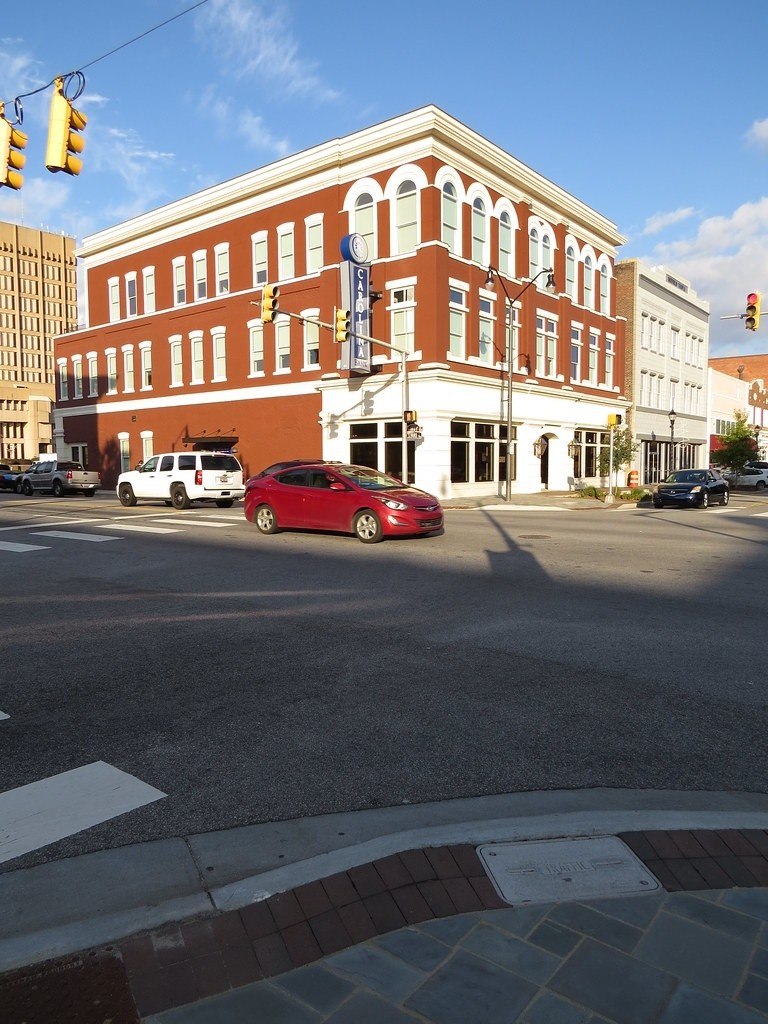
[244,463,445,544]
[722,467,768,491]
[0,464,21,492]
[743,459,768,486]
[653,468,730,509]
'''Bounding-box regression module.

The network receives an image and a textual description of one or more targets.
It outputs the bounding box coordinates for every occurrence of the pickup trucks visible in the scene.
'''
[21,460,103,497]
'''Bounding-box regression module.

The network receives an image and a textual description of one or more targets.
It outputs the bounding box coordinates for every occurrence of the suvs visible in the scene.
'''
[116,449,247,510]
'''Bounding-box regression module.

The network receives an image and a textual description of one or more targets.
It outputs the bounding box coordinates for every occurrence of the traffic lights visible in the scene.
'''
[609,414,622,427]
[46,92,88,177]
[261,282,281,325]
[333,306,351,345]
[745,291,761,331]
[0,117,28,191]
[404,409,416,423]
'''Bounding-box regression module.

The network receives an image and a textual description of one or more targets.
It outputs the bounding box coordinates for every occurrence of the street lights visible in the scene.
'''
[484,265,557,502]
[668,408,677,475]
[754,425,762,460]
